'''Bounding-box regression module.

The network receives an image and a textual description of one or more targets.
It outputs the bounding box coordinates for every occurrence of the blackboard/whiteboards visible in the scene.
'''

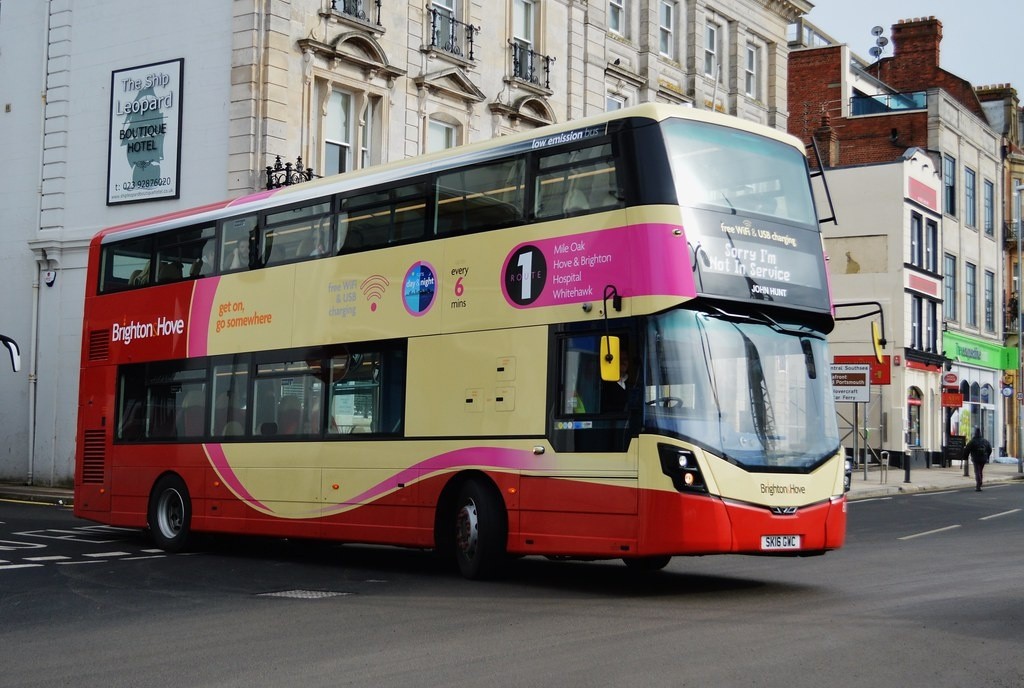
[946,436,966,460]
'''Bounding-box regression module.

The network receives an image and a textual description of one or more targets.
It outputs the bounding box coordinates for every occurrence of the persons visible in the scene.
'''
[963,428,992,492]
[599,351,644,415]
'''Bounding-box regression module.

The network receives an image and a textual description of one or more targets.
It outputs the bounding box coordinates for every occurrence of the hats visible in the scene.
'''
[975,428,981,436]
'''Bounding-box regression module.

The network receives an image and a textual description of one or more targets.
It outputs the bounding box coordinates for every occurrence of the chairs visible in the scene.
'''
[132,218,360,291]
[121,388,337,434]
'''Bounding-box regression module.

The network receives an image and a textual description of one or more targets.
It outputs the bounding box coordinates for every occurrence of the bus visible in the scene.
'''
[73,102,887,582]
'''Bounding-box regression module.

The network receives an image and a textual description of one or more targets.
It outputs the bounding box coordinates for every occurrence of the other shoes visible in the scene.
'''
[975,486,982,491]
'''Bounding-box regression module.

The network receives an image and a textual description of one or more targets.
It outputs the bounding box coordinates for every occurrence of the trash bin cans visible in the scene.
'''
[844,456,853,492]
